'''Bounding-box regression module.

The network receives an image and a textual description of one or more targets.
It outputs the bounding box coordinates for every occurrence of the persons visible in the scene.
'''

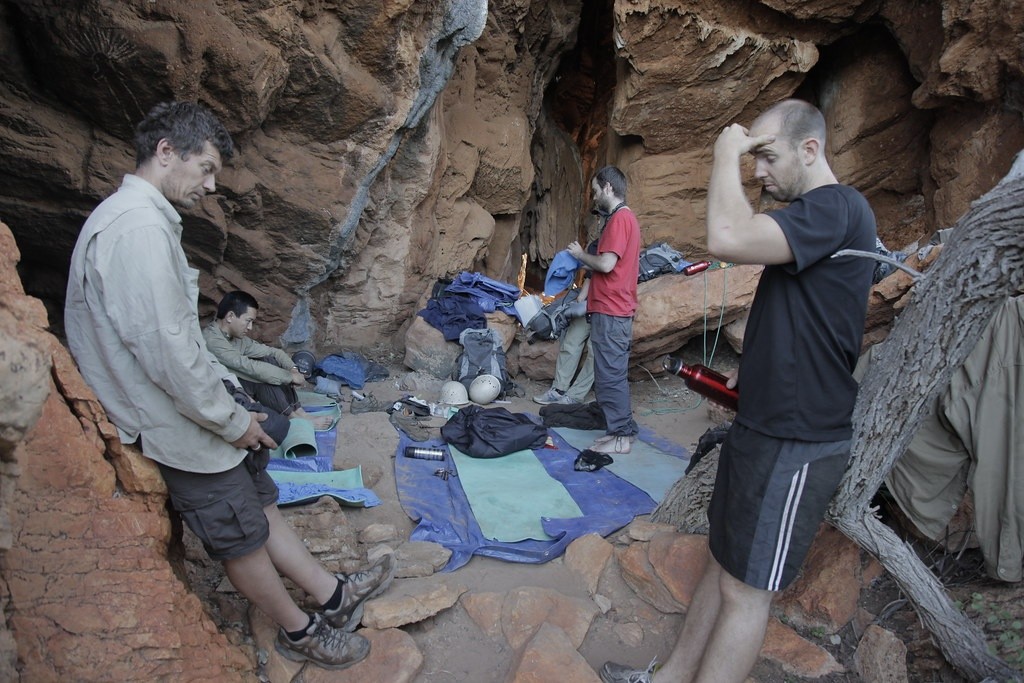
[533,207,607,405]
[566,167,640,454]
[600,98,876,683]
[203,291,334,431]
[64,102,397,671]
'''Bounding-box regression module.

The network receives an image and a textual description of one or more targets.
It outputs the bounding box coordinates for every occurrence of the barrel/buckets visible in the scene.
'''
[292,350,316,379]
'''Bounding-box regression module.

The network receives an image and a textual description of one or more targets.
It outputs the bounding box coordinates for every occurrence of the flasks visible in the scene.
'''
[662,353,738,413]
[404,446,445,461]
[683,260,712,275]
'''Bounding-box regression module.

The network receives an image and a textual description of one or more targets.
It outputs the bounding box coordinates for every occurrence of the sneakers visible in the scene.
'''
[533,385,565,404]
[558,395,580,405]
[350,391,393,414]
[324,553,397,633]
[391,410,429,442]
[600,655,663,683]
[275,612,371,670]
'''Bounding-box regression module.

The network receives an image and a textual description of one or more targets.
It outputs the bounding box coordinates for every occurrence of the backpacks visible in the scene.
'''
[637,240,684,284]
[452,328,514,400]
[526,287,581,345]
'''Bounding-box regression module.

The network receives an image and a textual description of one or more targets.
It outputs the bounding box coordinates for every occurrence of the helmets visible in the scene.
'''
[469,375,501,405]
[438,381,469,405]
